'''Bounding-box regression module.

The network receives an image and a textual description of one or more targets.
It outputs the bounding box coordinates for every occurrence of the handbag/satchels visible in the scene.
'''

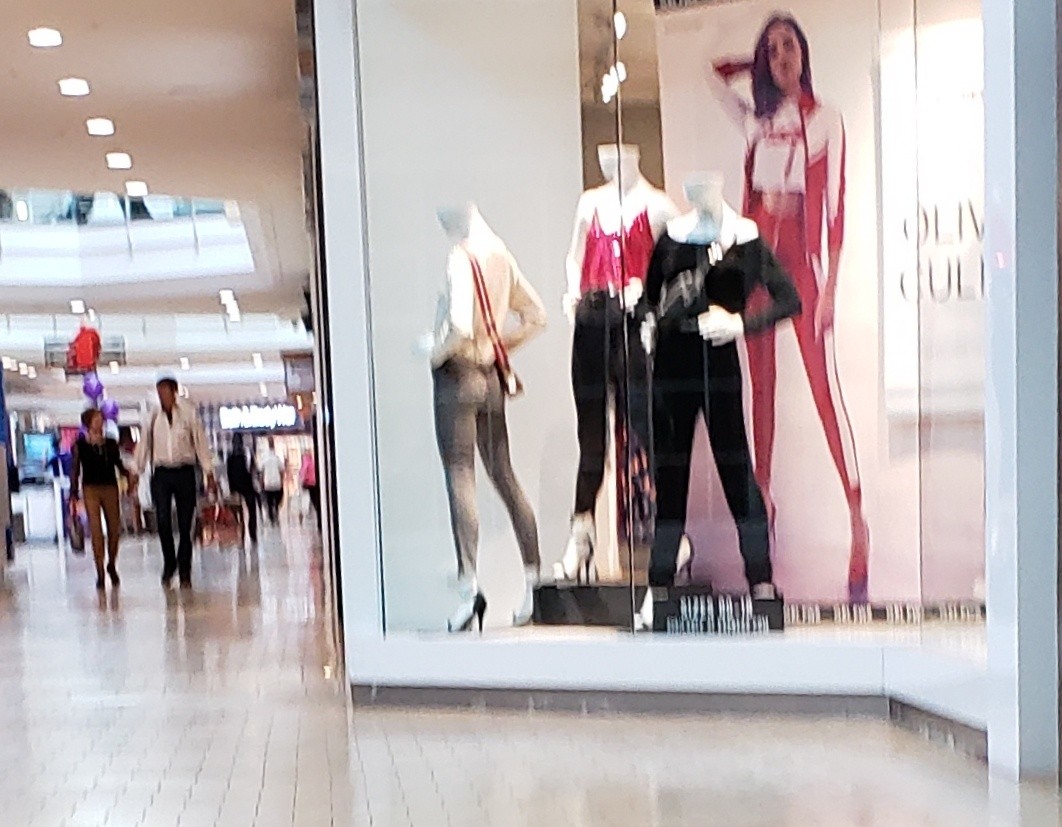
[66,498,83,550]
[495,359,524,398]
[190,485,240,547]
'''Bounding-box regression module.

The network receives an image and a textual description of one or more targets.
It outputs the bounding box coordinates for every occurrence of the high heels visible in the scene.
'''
[449,594,487,631]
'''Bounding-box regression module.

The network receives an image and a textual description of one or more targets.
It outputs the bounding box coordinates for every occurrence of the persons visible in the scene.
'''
[136,378,213,588]
[712,13,872,609]
[554,143,695,583]
[427,196,546,630]
[49,419,322,547]
[68,409,135,588]
[632,171,801,629]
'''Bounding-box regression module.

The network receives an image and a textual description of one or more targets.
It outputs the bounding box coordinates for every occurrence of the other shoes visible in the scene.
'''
[179,566,191,586]
[162,562,177,583]
[107,564,120,584]
[96,578,104,587]
